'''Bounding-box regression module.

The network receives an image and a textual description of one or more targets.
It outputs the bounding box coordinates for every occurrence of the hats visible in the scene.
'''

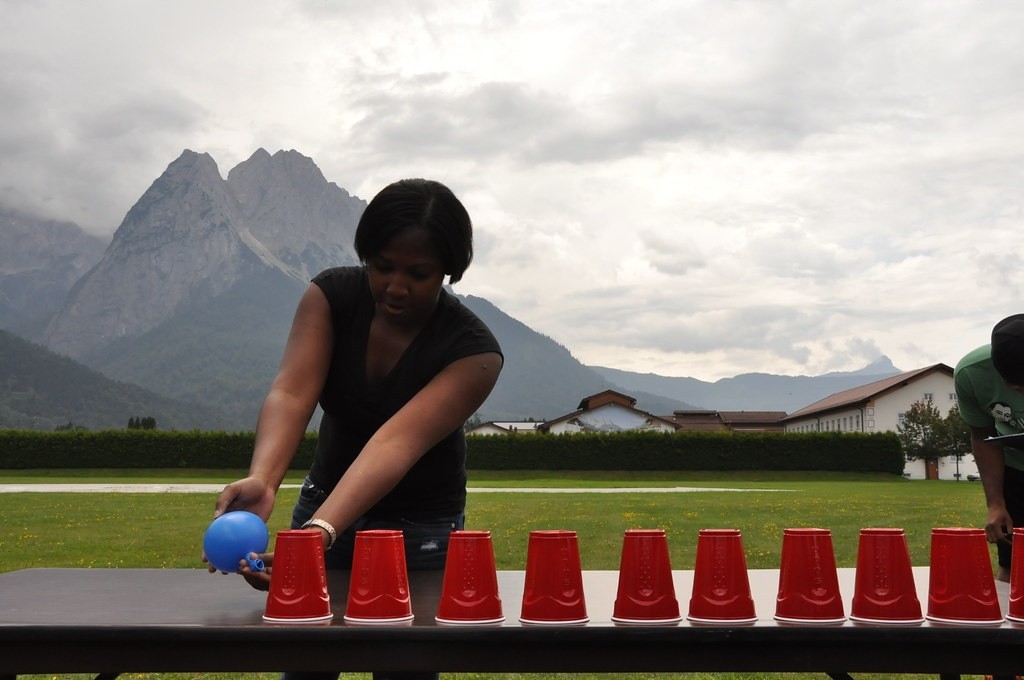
[990,313,1024,386]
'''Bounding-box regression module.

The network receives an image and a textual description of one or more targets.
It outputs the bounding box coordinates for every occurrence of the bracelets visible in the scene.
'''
[300,519,337,551]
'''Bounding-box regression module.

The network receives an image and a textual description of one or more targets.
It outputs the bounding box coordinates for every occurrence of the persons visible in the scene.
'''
[953,314,1024,585]
[202,179,507,680]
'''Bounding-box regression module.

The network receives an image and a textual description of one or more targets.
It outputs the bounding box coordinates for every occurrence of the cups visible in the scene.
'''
[519,529,590,623]
[773,528,847,623]
[1006,527,1024,622]
[925,528,1005,623]
[686,528,758,622]
[262,530,334,622]
[612,529,682,623]
[849,528,925,623]
[435,531,505,623]
[343,529,414,623]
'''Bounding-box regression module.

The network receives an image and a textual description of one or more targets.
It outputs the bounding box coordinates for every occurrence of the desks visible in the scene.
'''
[0,566,1024,680]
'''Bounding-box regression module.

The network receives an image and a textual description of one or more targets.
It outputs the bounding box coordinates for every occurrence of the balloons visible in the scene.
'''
[203,511,271,575]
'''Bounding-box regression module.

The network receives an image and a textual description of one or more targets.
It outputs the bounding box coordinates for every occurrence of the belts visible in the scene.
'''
[311,473,464,523]
